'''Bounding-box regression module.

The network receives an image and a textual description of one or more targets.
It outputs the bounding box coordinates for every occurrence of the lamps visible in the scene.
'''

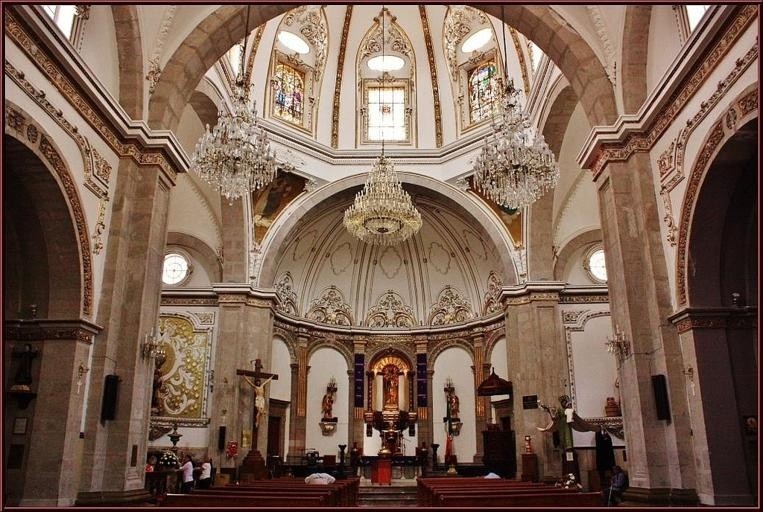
[190,3,280,200]
[343,6,423,246]
[142,324,168,361]
[472,4,559,213]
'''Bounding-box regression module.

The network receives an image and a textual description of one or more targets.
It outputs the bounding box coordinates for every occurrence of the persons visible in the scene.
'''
[243,374,274,428]
[10,343,40,385]
[484,467,501,479]
[549,400,575,452]
[199,457,211,488]
[605,466,629,505]
[179,454,194,493]
[305,465,335,485]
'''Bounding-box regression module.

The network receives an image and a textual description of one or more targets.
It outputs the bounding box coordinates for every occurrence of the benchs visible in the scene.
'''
[158,476,361,506]
[416,475,601,507]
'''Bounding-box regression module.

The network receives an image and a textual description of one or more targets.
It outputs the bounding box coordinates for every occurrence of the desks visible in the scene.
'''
[362,453,422,482]
